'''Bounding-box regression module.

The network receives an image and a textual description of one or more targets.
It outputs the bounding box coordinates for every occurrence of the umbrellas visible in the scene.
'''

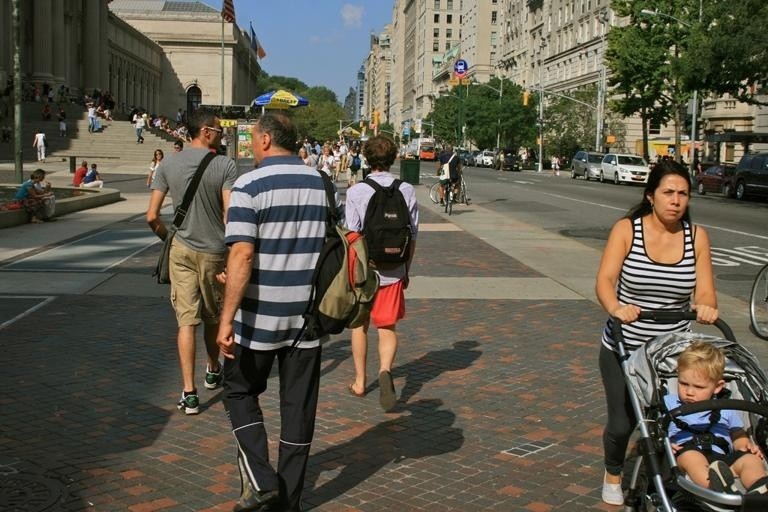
[250,89,309,115]
[337,127,360,139]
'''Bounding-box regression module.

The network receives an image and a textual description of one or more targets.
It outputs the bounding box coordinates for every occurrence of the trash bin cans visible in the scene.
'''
[400,158,420,185]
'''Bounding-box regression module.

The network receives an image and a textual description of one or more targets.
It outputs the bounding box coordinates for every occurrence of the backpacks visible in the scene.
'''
[363,187,414,268]
[350,156,361,173]
[303,219,381,341]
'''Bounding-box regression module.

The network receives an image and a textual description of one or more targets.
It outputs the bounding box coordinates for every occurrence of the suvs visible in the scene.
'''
[495,150,521,172]
[734,153,767,201]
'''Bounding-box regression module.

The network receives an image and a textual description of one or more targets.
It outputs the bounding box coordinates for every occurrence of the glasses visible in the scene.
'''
[174,146,180,149]
[155,153,162,157]
[200,125,224,135]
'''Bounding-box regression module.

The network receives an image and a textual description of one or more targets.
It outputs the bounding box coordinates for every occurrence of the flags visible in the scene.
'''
[221,0,235,23]
[249,21,266,60]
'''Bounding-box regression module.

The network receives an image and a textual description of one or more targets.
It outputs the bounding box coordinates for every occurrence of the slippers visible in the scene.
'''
[378,369,397,413]
[346,380,368,397]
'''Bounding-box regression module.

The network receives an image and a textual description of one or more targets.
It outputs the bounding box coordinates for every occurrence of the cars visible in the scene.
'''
[693,166,734,196]
[444,148,495,167]
[570,149,601,180]
[600,153,653,185]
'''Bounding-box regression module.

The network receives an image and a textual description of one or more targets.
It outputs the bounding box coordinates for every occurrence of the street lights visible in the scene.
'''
[442,93,468,148]
[522,83,603,150]
[470,75,503,150]
[640,8,700,187]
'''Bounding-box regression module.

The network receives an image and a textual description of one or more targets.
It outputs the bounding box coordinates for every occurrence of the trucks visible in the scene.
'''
[411,138,436,160]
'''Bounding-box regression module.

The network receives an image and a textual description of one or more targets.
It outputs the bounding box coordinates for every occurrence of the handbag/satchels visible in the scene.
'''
[152,226,173,285]
[439,163,451,185]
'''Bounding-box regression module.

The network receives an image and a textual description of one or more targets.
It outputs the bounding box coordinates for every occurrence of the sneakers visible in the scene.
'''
[232,482,280,511]
[601,468,626,506]
[174,387,200,417]
[203,360,226,391]
[706,459,742,496]
[744,479,768,499]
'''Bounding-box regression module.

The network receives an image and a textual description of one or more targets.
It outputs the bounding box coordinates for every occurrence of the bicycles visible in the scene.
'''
[750,263,768,341]
[441,181,454,214]
[429,166,469,204]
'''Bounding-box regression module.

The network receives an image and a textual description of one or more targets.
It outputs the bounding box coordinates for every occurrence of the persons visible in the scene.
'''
[550,155,561,176]
[344,134,419,412]
[434,143,462,206]
[655,339,768,499]
[16,168,59,222]
[146,148,164,192]
[145,108,239,415]
[520,151,527,167]
[594,157,720,507]
[295,130,372,188]
[498,149,505,171]
[214,112,340,512]
[655,151,704,177]
[79,163,104,189]
[73,161,88,187]
[0,77,193,164]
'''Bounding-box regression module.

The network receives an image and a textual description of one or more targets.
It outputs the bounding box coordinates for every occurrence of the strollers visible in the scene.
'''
[612,310,767,512]
